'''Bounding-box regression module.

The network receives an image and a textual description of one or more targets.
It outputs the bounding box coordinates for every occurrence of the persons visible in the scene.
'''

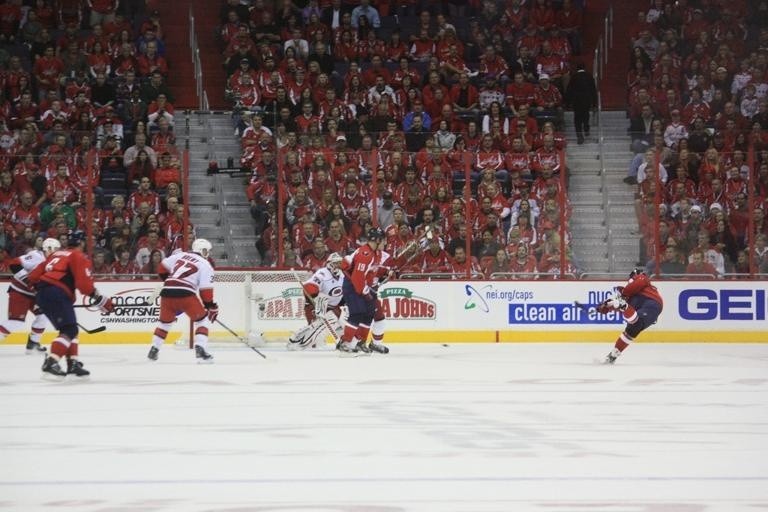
[336,227,385,358]
[289,252,349,348]
[625,0,768,281]
[148,239,218,361]
[1,0,194,280]
[28,229,113,374]
[340,233,418,353]
[596,267,662,363]
[0,238,60,352]
[218,1,579,279]
[565,63,594,146]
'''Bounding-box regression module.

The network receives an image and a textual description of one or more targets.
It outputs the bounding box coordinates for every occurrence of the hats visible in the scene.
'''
[538,74,550,81]
[690,205,702,213]
[336,135,347,142]
[240,58,250,65]
[670,109,680,116]
[716,67,728,73]
[709,204,722,211]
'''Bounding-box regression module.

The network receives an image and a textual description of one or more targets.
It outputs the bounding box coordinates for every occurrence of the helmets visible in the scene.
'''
[40,237,60,251]
[366,227,387,242]
[67,230,87,251]
[191,239,211,259]
[327,253,343,269]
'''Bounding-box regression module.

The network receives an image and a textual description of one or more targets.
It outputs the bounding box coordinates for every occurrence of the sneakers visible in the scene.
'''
[336,341,349,352]
[607,353,613,362]
[369,340,389,353]
[353,342,370,353]
[148,346,159,360]
[195,346,214,359]
[67,359,90,376]
[25,341,48,352]
[41,358,67,376]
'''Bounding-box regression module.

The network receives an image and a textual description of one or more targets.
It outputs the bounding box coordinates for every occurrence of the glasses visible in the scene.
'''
[516,124,528,128]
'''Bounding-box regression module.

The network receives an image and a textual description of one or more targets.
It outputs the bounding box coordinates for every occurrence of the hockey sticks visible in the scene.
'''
[212,316,265,359]
[72,296,103,307]
[574,273,658,315]
[75,322,105,334]
[289,266,341,345]
